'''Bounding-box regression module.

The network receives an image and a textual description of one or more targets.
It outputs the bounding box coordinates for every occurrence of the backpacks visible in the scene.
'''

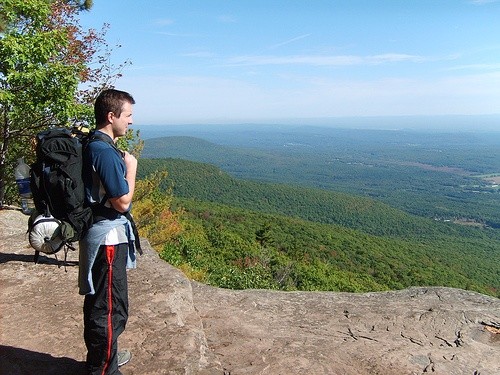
[28,126,121,254]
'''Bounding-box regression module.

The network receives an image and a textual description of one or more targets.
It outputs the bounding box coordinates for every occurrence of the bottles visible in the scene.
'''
[14,157,37,214]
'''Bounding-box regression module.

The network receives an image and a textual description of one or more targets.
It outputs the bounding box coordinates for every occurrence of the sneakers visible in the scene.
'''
[117,350,131,366]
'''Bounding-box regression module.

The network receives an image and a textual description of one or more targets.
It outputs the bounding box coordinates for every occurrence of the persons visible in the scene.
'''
[76,88,145,375]
